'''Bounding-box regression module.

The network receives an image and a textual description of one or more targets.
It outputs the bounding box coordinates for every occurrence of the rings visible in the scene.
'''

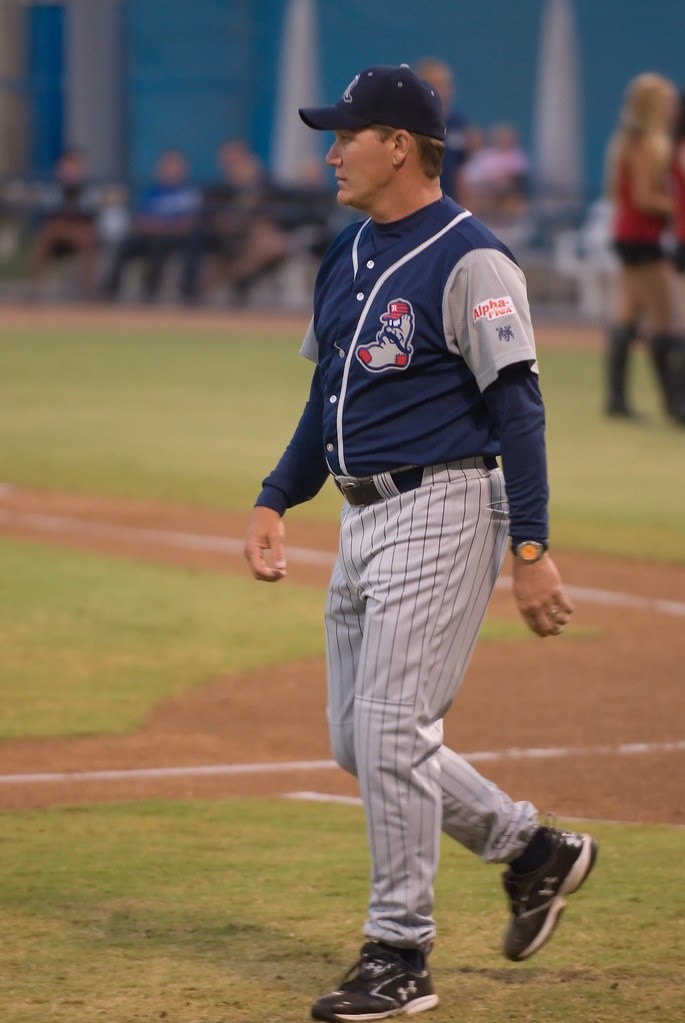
[549,609,560,616]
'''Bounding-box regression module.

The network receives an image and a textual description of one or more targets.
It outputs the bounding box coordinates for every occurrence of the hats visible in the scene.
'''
[298,63,447,141]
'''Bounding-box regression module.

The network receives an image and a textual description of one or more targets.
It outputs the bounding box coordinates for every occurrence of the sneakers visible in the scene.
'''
[502,812,597,963]
[311,941,440,1022]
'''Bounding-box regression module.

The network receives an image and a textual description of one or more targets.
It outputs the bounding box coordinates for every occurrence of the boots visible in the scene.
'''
[604,325,630,415]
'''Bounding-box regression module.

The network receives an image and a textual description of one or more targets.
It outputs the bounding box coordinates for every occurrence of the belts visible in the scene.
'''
[334,455,500,506]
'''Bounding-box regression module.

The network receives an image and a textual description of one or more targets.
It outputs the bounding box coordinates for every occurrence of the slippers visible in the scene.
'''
[652,334,685,426]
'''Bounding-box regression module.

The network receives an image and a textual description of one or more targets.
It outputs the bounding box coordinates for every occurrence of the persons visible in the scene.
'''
[24,58,538,307]
[242,63,597,1023]
[603,70,685,429]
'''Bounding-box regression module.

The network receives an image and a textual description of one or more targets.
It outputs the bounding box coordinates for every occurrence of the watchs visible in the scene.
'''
[511,540,547,563]
